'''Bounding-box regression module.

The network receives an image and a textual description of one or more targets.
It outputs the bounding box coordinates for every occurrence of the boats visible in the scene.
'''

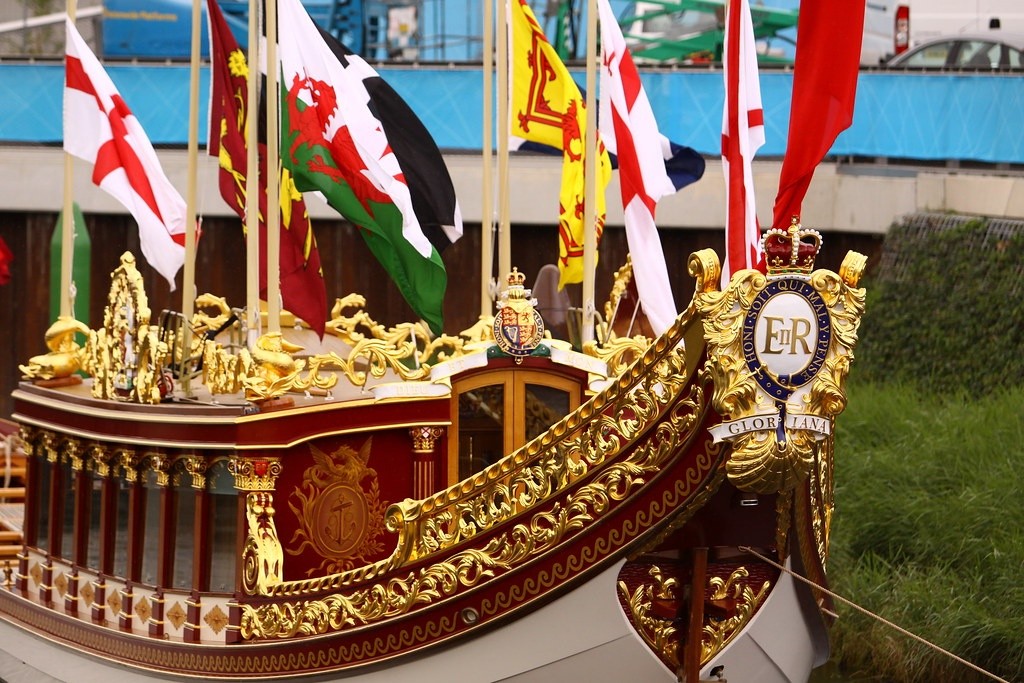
[1,0,870,682]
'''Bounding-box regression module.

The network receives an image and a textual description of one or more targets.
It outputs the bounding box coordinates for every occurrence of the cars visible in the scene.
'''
[884,26,1024,74]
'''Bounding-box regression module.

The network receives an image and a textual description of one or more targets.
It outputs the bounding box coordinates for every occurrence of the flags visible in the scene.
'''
[62,16,202,293]
[721,0,767,292]
[505,0,613,290]
[772,0,866,230]
[600,0,707,339]
[256,0,463,369]
[207,0,327,344]
